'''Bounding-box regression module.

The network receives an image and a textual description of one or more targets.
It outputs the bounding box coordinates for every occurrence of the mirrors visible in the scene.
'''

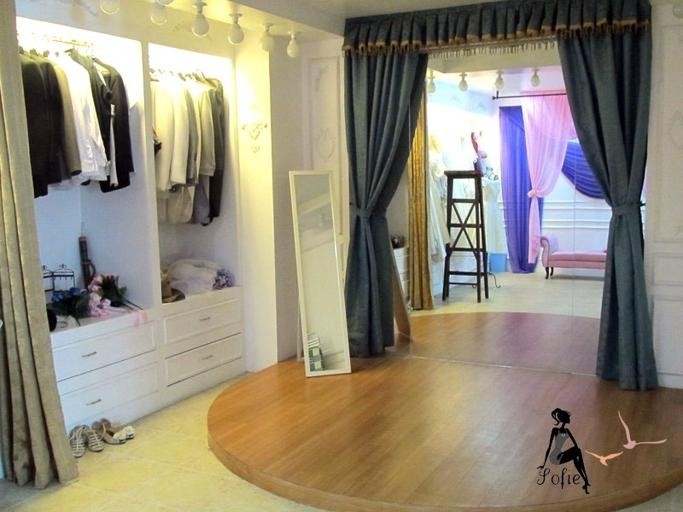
[288,168,353,378]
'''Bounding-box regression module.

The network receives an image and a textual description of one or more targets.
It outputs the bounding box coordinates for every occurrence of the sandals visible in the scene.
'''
[70,419,135,457]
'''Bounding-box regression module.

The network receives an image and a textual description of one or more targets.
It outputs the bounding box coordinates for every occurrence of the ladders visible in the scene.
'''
[443,170,489,303]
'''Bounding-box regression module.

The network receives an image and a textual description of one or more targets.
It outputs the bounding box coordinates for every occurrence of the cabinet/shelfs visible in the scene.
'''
[16,1,161,446]
[391,245,410,299]
[145,1,247,405]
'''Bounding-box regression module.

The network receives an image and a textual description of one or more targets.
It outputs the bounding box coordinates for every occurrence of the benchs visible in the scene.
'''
[539,232,609,278]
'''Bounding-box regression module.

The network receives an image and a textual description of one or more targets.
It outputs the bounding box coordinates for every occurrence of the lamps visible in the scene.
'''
[191,2,208,35]
[286,30,301,58]
[426,71,436,93]
[530,68,540,87]
[98,1,121,14]
[226,11,245,45]
[260,22,277,52]
[459,72,467,91]
[150,1,173,26]
[495,70,503,88]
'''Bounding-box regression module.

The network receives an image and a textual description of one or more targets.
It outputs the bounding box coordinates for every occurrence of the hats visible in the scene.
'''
[161,274,180,303]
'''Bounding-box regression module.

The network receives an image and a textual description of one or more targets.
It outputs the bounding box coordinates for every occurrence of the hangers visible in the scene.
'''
[149,64,216,88]
[17,31,96,60]
